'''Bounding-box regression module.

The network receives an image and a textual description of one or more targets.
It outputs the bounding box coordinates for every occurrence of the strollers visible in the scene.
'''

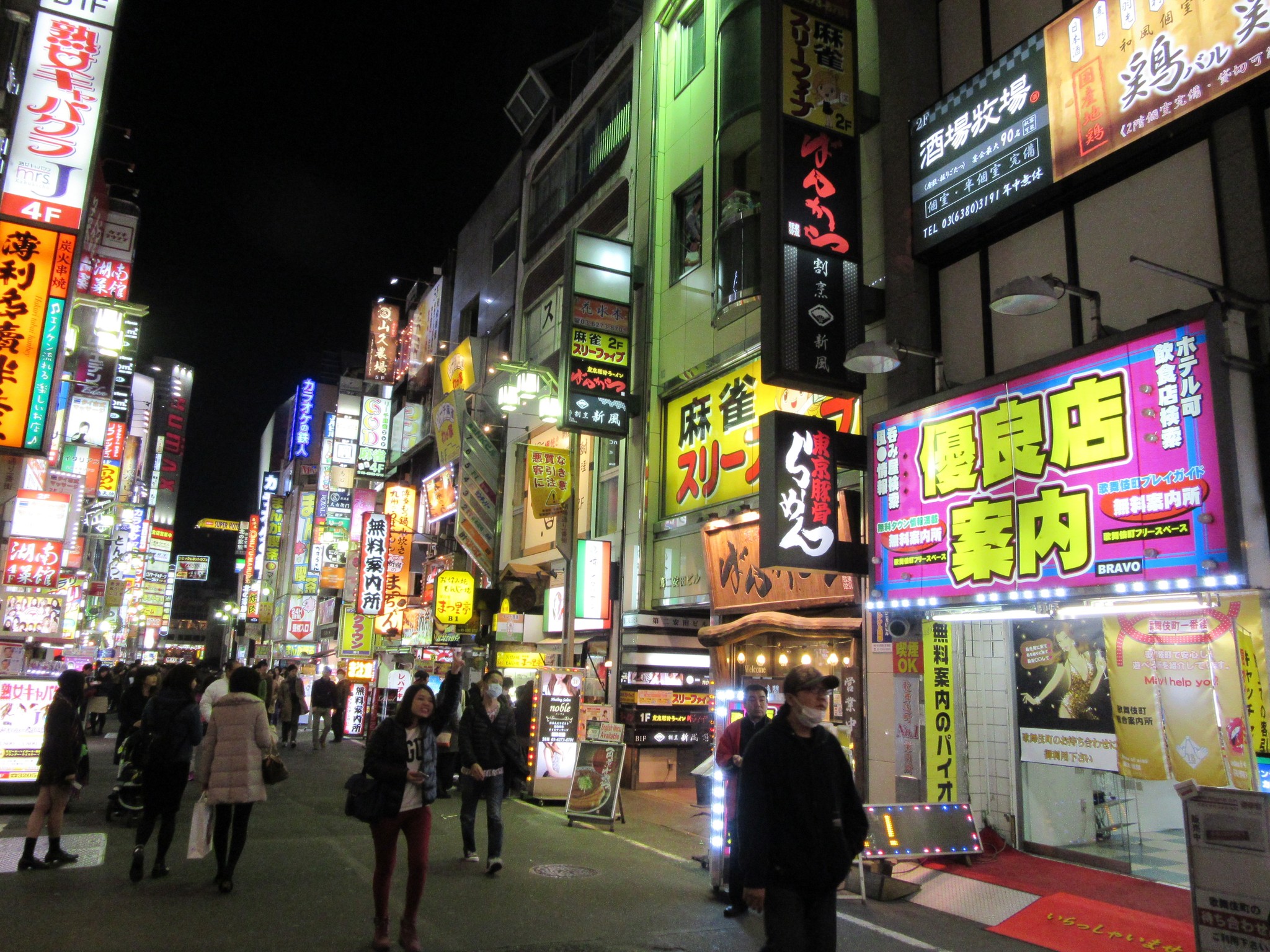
[106,727,147,828]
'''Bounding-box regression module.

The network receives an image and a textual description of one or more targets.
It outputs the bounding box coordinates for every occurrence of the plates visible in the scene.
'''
[567,767,611,814]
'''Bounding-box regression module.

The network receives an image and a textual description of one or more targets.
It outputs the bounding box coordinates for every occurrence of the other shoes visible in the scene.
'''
[373,924,391,951]
[44,850,79,862]
[320,741,326,748]
[152,865,168,877]
[188,773,195,781]
[218,875,234,892]
[129,846,144,881]
[291,741,296,748]
[466,852,479,861]
[313,743,318,749]
[18,857,49,871]
[331,738,341,742]
[487,858,501,873]
[399,927,422,951]
[723,903,746,916]
[281,741,288,747]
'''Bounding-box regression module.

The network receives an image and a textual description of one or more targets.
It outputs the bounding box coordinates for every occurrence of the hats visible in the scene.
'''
[785,664,841,691]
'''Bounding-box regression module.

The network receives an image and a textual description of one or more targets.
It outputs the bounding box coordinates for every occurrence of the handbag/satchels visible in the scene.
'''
[343,774,382,824]
[188,792,213,859]
[262,736,288,785]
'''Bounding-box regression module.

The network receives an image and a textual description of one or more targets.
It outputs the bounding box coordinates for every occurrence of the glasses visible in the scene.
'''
[803,688,831,696]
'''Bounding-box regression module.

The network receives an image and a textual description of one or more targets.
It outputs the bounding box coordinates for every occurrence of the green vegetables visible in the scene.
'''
[578,775,592,790]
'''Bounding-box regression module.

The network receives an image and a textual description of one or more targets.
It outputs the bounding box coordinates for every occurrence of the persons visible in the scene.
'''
[715,665,869,952]
[17,657,534,952]
[0,596,61,672]
[544,672,580,696]
[685,193,701,257]
[542,741,571,778]
[71,422,92,445]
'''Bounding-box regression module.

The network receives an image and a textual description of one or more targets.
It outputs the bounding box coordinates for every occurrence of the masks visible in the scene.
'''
[484,683,502,698]
[792,697,825,728]
